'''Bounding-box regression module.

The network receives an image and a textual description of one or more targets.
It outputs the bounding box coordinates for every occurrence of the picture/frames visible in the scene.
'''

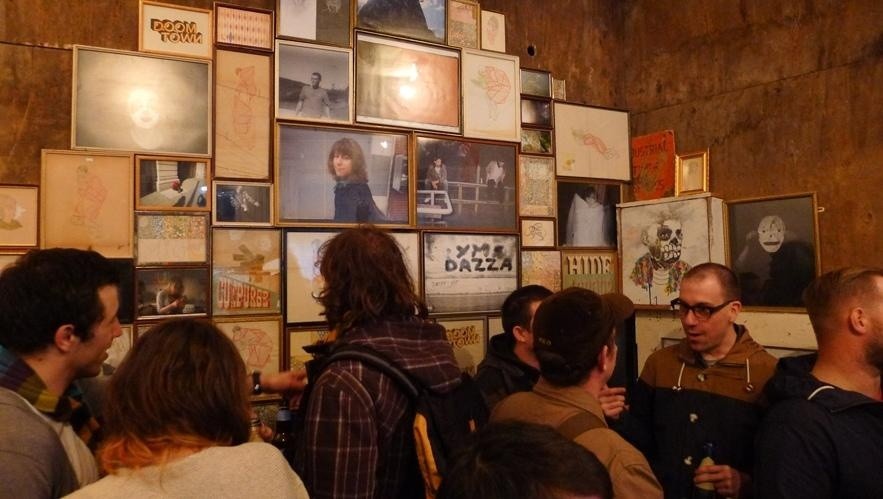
[0,0,823,438]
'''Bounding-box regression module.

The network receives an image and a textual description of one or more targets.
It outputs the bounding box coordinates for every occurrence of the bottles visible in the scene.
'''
[695,444,715,490]
[270,399,296,469]
[245,417,264,443]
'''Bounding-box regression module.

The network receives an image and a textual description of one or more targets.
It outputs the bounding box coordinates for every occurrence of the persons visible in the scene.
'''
[752,266,882,498]
[623,263,779,499]
[487,285,665,499]
[292,228,463,498]
[424,156,449,203]
[61,317,309,499]
[328,137,387,222]
[295,73,332,118]
[0,247,306,498]
[154,276,185,313]
[434,421,614,499]
[469,285,629,428]
[484,157,508,203]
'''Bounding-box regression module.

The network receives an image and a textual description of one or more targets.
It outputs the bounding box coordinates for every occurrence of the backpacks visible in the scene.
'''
[306,345,492,499]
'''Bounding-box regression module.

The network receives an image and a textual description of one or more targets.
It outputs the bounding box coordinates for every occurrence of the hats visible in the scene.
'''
[530,286,636,353]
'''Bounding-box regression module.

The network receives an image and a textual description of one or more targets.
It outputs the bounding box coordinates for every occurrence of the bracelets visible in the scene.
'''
[253,371,262,395]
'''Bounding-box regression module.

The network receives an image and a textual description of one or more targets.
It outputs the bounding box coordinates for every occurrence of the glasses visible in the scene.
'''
[671,298,741,319]
